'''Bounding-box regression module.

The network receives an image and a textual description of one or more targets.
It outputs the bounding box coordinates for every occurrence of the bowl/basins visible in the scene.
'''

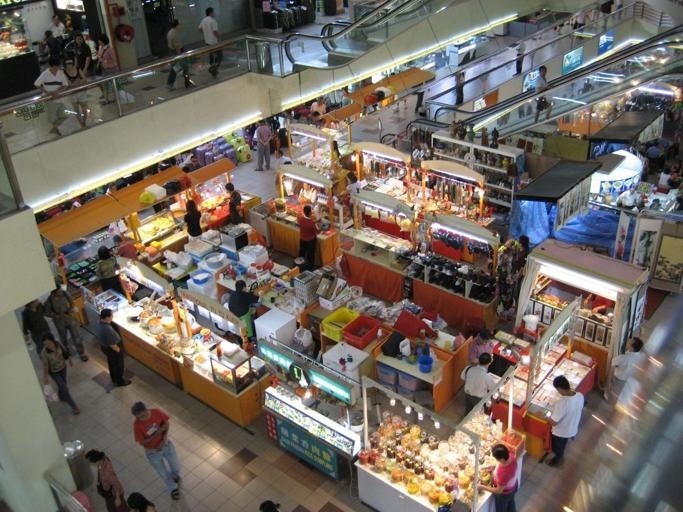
[204,253,225,268]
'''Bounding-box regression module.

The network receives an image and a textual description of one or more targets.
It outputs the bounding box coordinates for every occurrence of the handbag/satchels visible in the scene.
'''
[62,350,68,359]
[97,483,113,500]
[114,264,120,274]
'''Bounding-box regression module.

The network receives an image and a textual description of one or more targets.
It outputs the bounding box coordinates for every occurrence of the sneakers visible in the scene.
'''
[80,352,88,360]
[547,459,563,469]
[114,380,131,385]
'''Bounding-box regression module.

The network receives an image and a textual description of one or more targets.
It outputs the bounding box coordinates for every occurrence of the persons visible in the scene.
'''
[96,307,132,386]
[251,120,273,171]
[610,336,648,401]
[39,331,81,414]
[182,200,202,241]
[553,0,625,39]
[259,498,282,512]
[44,283,89,361]
[543,374,585,469]
[295,204,320,268]
[308,96,326,117]
[197,7,222,79]
[228,279,264,344]
[411,86,430,113]
[94,245,127,299]
[272,149,291,171]
[21,298,73,362]
[477,444,521,512]
[129,399,185,501]
[126,490,158,512]
[84,447,127,512]
[186,155,203,171]
[533,65,555,121]
[614,132,683,211]
[34,14,119,140]
[142,166,161,215]
[624,88,647,113]
[225,182,244,224]
[305,112,321,129]
[512,40,528,78]
[165,17,196,91]
[111,235,141,263]
[452,69,468,103]
[296,181,320,204]
[458,350,501,422]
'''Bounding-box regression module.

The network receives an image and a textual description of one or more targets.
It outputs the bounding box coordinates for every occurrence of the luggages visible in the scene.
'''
[196,132,252,166]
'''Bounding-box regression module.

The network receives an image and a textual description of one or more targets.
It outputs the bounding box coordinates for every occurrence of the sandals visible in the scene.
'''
[171,476,179,500]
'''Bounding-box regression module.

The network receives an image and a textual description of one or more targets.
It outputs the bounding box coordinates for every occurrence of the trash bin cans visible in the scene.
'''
[62,440,93,491]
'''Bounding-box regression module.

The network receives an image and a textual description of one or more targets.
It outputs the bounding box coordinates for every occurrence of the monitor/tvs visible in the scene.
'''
[51,1,87,15]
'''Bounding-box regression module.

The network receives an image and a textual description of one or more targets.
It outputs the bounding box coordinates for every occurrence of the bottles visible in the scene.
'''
[423,333,429,355]
[247,263,262,284]
[449,120,498,149]
[414,336,423,359]
[432,138,513,203]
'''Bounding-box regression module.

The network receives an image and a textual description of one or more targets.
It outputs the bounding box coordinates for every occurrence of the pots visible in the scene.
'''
[147,316,163,334]
[138,310,152,327]
